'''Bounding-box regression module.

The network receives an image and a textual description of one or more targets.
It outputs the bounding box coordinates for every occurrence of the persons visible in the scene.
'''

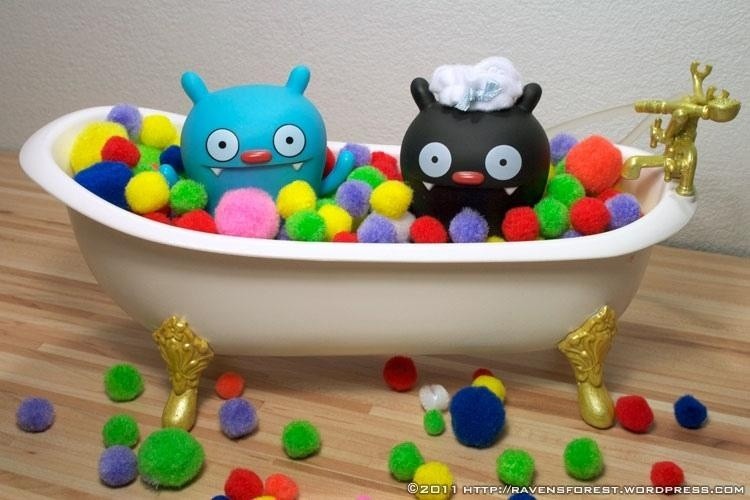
[16,55,743,500]
